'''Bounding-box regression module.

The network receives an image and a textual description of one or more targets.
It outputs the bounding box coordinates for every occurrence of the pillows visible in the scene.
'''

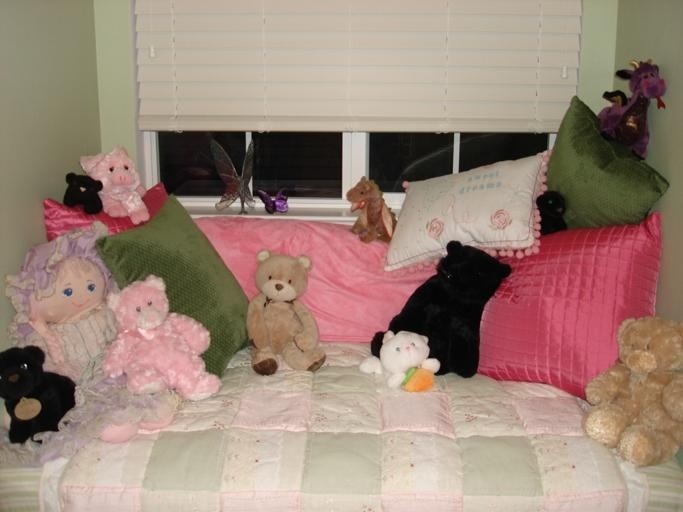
[477,210,663,401]
[382,150,551,276]
[96,194,252,377]
[547,95,669,227]
[43,181,167,242]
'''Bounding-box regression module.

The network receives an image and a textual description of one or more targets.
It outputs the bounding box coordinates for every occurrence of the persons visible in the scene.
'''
[1,227,182,463]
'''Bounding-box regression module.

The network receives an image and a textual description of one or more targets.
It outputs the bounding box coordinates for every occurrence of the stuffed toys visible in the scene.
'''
[586,316,683,465]
[64,172,103,215]
[79,144,150,224]
[534,191,568,235]
[104,274,223,401]
[243,249,327,375]
[347,176,398,242]
[362,329,441,390]
[597,58,666,160]
[370,240,511,378]
[0,345,76,443]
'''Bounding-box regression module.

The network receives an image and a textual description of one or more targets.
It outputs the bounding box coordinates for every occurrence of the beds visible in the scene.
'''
[1,216,683,511]
